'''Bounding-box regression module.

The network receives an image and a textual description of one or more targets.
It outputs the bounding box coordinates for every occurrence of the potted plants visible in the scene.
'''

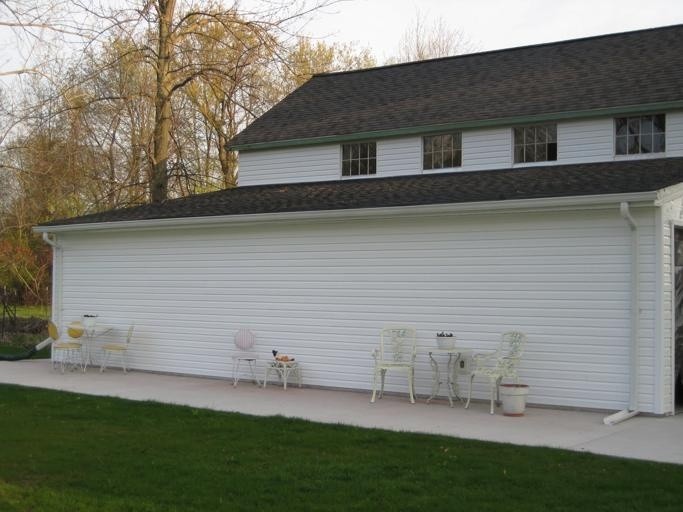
[436,330,454,350]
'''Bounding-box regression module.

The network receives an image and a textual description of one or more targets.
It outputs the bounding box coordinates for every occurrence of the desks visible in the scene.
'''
[262,359,302,390]
[415,344,472,407]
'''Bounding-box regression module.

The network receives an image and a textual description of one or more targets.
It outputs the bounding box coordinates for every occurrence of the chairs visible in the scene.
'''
[464,328,528,415]
[228,327,260,388]
[42,310,135,380]
[367,323,417,404]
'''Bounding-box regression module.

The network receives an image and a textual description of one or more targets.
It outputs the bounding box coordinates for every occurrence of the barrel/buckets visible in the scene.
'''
[83,315,97,326]
[437,335,456,350]
